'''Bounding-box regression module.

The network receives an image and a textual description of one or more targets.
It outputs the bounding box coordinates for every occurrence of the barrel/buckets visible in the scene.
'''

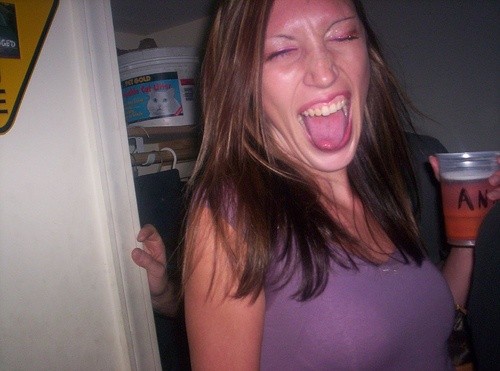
[118,45,206,129]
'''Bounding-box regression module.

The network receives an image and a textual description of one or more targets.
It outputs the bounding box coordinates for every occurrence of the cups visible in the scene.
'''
[434,150,500,246]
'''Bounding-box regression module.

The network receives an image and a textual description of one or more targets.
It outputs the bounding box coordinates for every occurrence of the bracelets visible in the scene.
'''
[455,302,467,314]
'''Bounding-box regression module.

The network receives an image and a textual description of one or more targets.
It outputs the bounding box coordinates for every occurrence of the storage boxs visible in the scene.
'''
[117,44,203,126]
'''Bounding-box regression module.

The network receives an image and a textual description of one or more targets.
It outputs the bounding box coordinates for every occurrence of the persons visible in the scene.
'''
[180,0,500,370]
[132,82,474,371]
[464,199,500,371]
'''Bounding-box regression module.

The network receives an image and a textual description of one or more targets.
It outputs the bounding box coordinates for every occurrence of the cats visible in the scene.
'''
[146,87,181,118]
